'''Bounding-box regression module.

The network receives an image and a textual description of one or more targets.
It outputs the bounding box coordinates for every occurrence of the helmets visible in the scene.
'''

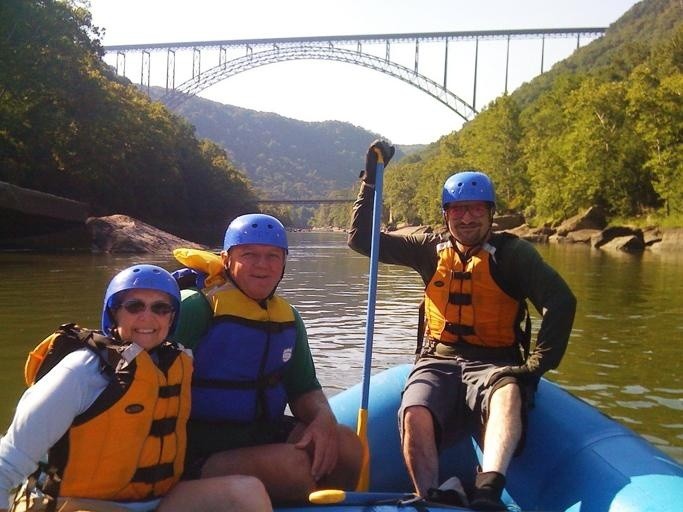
[441,172,496,209]
[224,214,289,256]
[102,264,182,344]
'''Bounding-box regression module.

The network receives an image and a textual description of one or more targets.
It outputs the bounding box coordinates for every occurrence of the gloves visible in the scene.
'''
[488,364,529,385]
[358,141,394,185]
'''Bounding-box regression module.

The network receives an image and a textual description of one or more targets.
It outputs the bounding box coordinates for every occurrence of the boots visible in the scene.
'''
[471,466,505,511]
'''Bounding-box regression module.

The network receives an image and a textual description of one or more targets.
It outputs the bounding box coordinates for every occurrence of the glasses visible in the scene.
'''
[449,204,486,220]
[120,302,176,314]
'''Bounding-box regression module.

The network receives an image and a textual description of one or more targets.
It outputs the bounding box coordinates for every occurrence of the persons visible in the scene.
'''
[0,264,277,512]
[347,141,577,512]
[166,213,364,506]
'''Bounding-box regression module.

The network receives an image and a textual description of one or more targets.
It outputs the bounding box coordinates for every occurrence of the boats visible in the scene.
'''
[283,363,683,512]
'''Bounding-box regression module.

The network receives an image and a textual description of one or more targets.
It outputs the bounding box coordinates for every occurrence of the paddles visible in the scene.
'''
[355,148,385,493]
